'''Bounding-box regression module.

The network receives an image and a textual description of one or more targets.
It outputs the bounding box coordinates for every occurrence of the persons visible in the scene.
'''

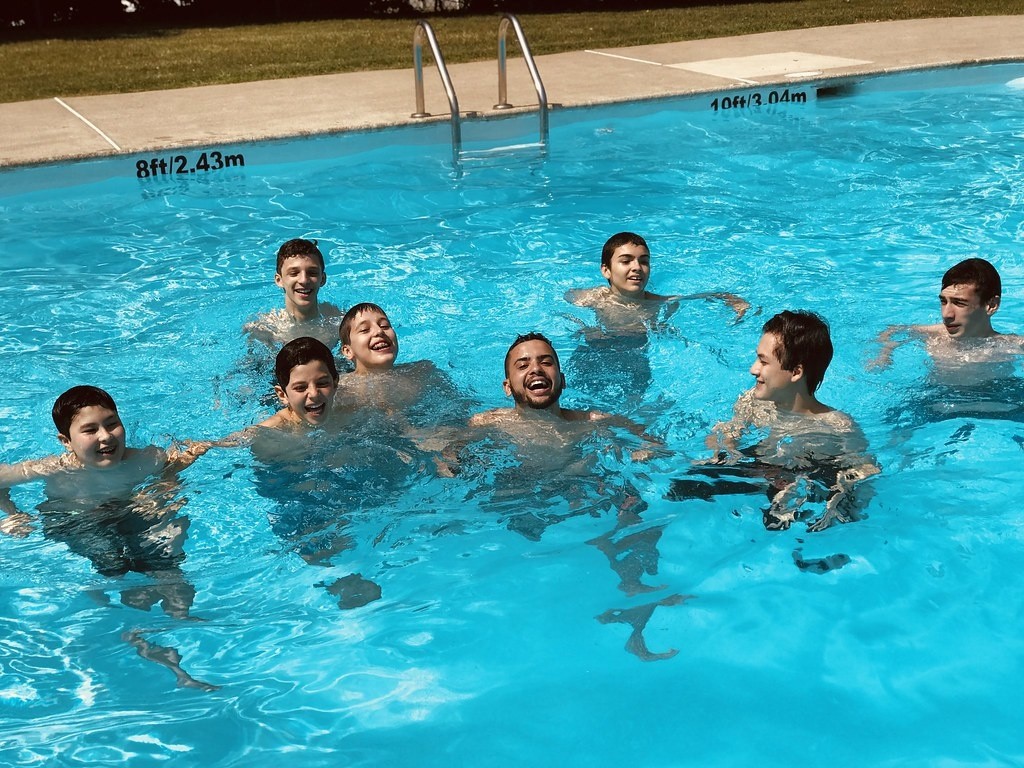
[599,232,676,302]
[255,239,399,438]
[915,258,1024,339]
[750,309,833,416]
[29,385,169,470]
[465,331,612,442]
